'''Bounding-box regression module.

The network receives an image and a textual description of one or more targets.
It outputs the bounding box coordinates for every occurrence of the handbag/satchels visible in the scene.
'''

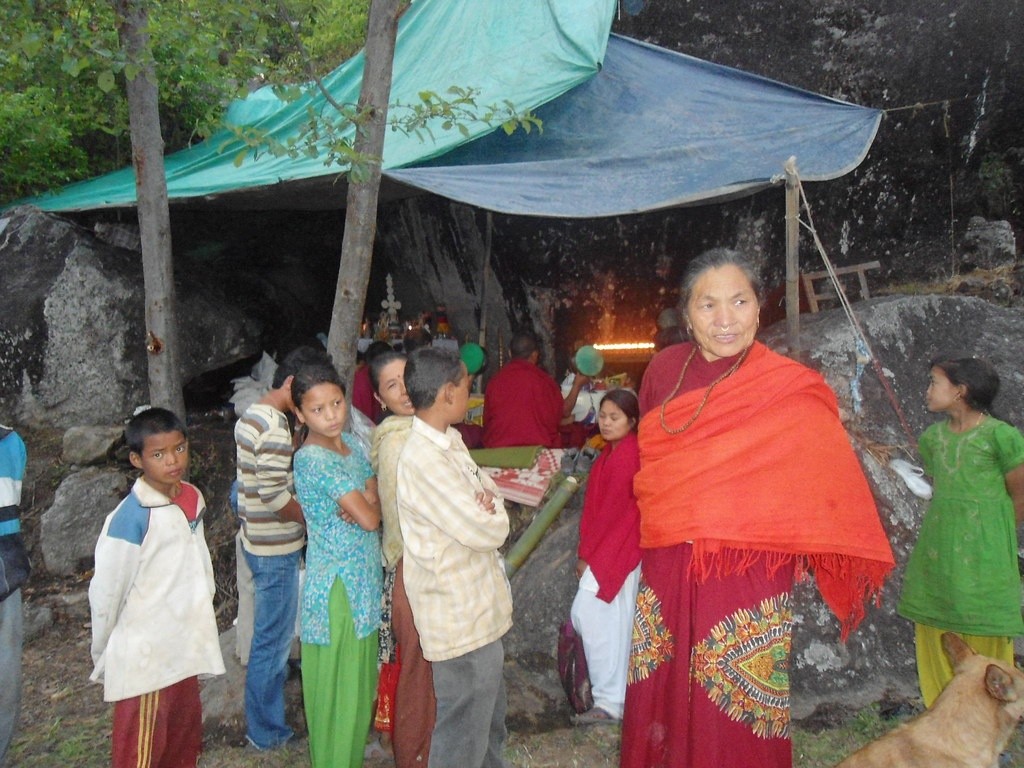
[557,622,594,713]
[373,640,401,731]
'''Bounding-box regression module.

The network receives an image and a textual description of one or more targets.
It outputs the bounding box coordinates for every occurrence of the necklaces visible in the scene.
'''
[660,345,747,435]
[976,413,983,426]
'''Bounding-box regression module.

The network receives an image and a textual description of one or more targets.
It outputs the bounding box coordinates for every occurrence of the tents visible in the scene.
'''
[0,0,883,217]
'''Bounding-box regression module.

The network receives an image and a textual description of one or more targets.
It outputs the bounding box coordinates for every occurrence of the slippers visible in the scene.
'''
[363,738,395,763]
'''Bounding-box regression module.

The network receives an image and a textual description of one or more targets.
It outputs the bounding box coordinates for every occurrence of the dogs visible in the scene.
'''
[831,630,1023,768]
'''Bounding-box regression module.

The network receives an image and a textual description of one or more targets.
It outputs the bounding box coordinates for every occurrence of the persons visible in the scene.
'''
[481,332,588,450]
[570,388,642,724]
[619,246,896,768]
[290,365,381,768]
[395,345,514,768]
[234,345,339,752]
[88,408,226,768]
[351,326,433,421]
[370,351,437,768]
[560,351,607,424]
[896,358,1024,707]
[0,424,29,759]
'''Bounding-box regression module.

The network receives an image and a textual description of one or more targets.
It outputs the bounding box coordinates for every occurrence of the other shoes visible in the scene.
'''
[570,706,619,726]
